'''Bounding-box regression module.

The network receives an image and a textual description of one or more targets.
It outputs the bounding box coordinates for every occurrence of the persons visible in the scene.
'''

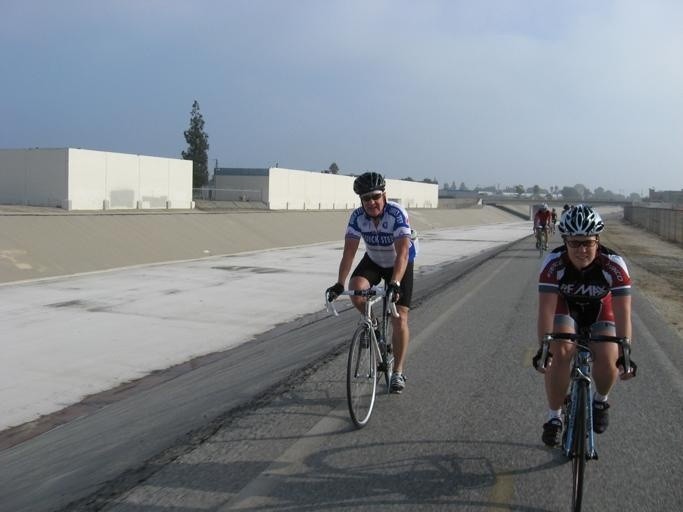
[549,209,556,234]
[325,170,418,392]
[531,204,638,447]
[561,204,569,216]
[532,203,552,251]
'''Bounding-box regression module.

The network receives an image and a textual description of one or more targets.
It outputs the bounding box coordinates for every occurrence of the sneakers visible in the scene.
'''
[592,392,610,433]
[391,374,406,391]
[360,332,369,348]
[542,418,562,445]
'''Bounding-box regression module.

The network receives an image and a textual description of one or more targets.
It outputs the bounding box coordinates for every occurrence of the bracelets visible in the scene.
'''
[387,278,402,288]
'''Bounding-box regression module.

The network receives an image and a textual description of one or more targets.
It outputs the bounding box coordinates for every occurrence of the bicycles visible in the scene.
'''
[532,329,637,512]
[550,219,556,236]
[322,284,395,429]
[532,225,550,259]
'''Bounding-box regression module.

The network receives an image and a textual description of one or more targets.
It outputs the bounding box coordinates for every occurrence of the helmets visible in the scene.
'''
[540,203,547,213]
[559,205,604,236]
[354,173,385,194]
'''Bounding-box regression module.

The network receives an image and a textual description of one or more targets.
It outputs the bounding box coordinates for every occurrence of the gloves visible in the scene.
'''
[327,283,344,302]
[386,283,400,303]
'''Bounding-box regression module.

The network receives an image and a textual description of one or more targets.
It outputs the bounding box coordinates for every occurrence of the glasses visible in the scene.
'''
[362,193,381,200]
[566,240,599,248]
